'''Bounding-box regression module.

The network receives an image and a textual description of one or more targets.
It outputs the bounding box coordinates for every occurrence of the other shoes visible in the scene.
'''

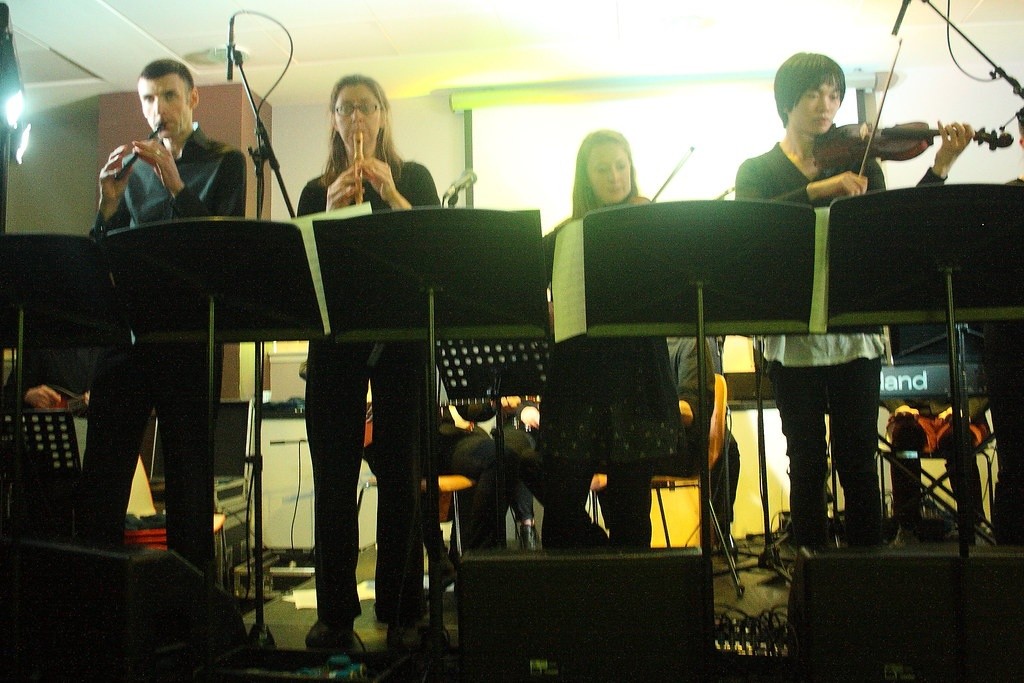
[385,621,450,652]
[712,533,739,559]
[520,524,540,555]
[849,520,899,550]
[913,516,953,543]
[305,620,354,649]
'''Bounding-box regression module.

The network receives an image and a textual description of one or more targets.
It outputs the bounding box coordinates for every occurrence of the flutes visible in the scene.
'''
[352,130,366,207]
[115,123,164,180]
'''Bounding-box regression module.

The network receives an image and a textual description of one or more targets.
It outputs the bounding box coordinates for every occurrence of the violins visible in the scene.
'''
[627,196,656,205]
[811,121,1014,171]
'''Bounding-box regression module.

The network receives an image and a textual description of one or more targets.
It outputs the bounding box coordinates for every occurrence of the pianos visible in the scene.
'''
[878,355,997,551]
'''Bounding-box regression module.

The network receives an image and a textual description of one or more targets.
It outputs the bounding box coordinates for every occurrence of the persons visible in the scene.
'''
[735,52,976,553]
[502,332,741,558]
[0,343,109,416]
[80,59,247,589]
[291,75,440,654]
[530,129,683,551]
[351,377,498,622]
[878,395,989,552]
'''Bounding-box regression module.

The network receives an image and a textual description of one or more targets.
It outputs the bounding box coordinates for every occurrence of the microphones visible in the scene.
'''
[891,0,911,36]
[443,170,478,199]
[226,18,234,80]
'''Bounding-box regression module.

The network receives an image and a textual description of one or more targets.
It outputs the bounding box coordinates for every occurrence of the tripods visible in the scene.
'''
[692,333,795,585]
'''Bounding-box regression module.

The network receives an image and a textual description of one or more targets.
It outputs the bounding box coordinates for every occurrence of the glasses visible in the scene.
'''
[335,103,378,117]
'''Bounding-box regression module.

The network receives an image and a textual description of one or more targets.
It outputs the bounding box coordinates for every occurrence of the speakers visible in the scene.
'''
[0,537,253,683]
[788,545,1024,683]
[458,547,710,683]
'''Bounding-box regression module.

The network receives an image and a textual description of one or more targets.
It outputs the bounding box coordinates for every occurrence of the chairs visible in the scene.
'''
[876,429,997,543]
[358,372,744,595]
